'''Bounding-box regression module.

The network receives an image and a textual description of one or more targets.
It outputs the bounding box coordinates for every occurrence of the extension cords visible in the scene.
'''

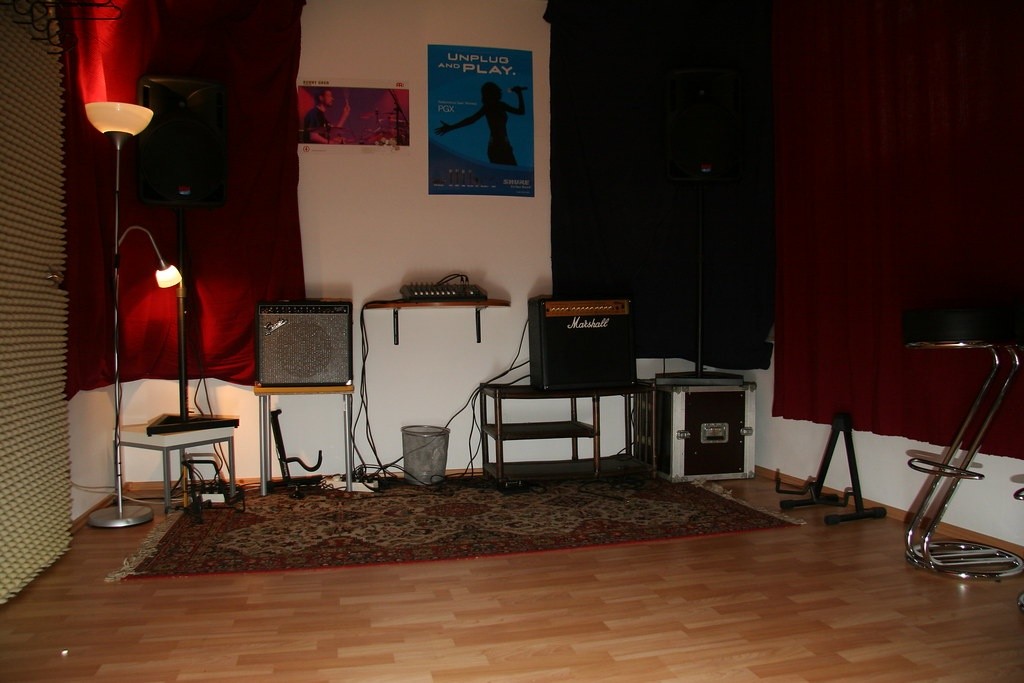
[331,475,379,492]
[190,492,226,503]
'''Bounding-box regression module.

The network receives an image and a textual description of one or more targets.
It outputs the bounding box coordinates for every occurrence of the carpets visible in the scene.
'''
[102,472,807,583]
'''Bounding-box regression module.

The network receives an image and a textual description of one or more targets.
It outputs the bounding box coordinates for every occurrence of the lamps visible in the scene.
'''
[70,0,183,529]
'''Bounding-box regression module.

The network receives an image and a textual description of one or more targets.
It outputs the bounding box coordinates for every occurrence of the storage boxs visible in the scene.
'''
[634,378,758,483]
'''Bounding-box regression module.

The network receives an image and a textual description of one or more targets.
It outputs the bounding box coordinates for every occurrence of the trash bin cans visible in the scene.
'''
[398,424,449,486]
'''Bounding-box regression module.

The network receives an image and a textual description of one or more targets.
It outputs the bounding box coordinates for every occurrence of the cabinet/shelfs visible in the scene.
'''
[479,382,600,487]
[254,382,355,498]
[112,424,234,513]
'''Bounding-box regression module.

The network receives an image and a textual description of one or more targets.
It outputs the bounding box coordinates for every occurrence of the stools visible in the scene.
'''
[900,300,1024,583]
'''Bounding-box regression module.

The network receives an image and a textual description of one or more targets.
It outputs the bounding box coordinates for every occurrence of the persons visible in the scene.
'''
[303,85,352,144]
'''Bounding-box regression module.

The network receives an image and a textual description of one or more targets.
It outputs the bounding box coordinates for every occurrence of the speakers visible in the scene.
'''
[253,298,354,387]
[132,73,230,209]
[665,68,742,184]
[527,294,638,394]
